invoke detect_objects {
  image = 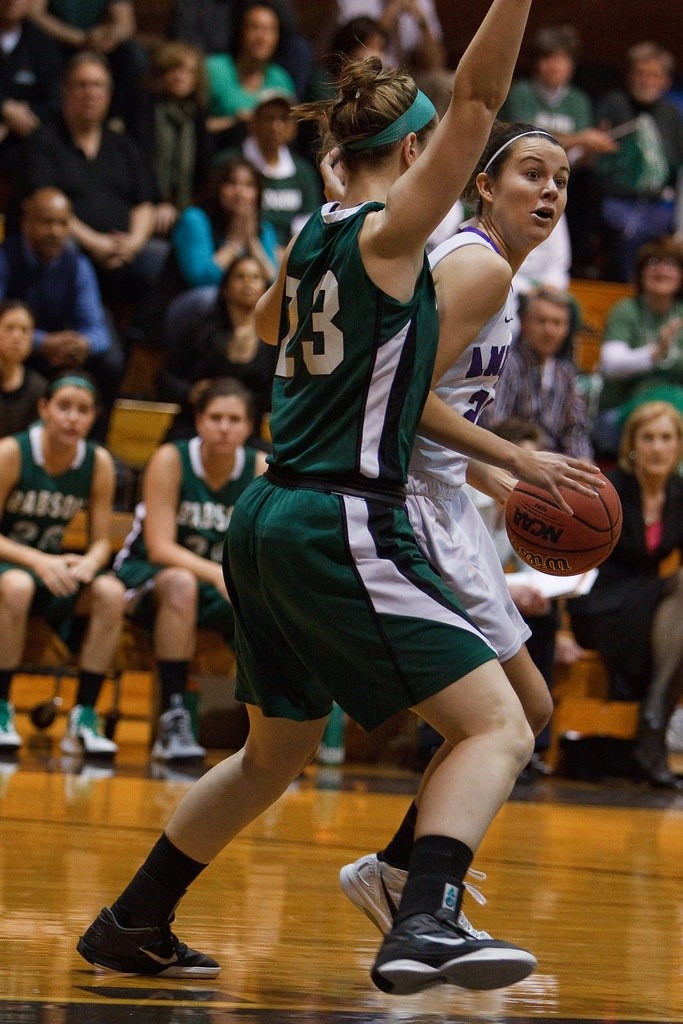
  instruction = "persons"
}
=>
[1,1,682,776]
[339,122,604,946]
[76,1,538,996]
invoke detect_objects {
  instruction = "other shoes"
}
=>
[628,709,676,789]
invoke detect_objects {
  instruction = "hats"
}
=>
[255,88,298,114]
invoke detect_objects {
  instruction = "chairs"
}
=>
[0,399,235,679]
[540,647,640,774]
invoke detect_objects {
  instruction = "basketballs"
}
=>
[505,464,622,578]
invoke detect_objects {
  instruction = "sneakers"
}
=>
[370,909,537,995]
[60,704,118,757]
[77,907,222,979]
[339,854,495,941]
[150,708,208,764]
[0,702,23,754]
